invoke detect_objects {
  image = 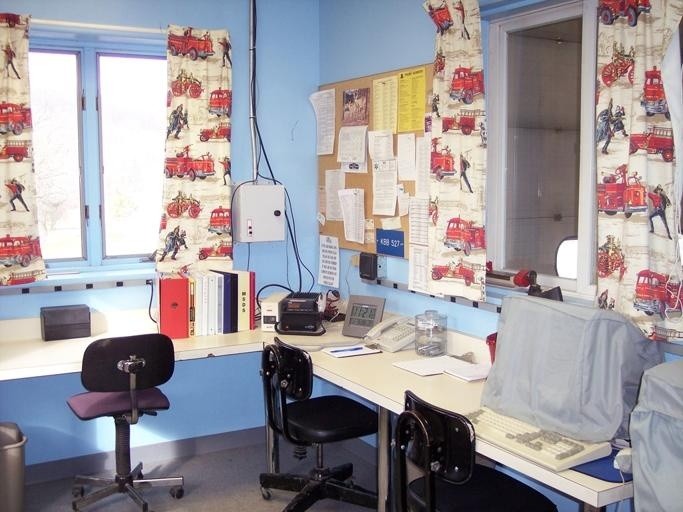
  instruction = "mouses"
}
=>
[614,447,633,472]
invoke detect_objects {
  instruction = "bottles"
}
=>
[415,309,447,356]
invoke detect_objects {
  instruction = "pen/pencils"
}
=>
[331,347,363,353]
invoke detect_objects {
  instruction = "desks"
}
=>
[0,293,632,512]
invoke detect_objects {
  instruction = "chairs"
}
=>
[67,332,186,511]
[254,329,377,512]
[391,384,561,512]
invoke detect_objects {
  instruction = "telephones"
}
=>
[364,316,416,353]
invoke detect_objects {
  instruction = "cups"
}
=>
[487,332,497,363]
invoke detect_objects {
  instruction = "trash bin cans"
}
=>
[0,422,27,512]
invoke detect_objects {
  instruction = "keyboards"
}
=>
[462,406,612,472]
